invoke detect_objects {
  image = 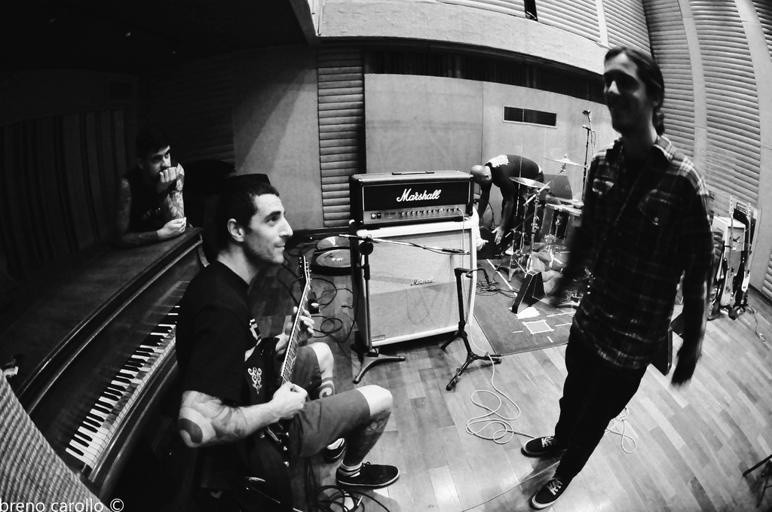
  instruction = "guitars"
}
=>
[239,254,311,469]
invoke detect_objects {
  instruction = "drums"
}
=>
[540,201,581,241]
[544,197,582,209]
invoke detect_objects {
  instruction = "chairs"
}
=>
[158,379,364,512]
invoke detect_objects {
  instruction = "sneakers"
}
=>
[335,462,399,488]
[323,439,345,463]
[531,478,569,509]
[523,435,567,456]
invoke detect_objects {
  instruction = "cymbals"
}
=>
[510,177,550,191]
[553,157,583,168]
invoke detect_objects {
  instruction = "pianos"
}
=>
[0,225,209,512]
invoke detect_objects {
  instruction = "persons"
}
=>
[470,153,544,245]
[111,130,191,248]
[175,175,400,494]
[519,46,713,509]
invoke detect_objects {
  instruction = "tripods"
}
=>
[441,268,501,390]
[494,164,566,282]
[339,234,470,383]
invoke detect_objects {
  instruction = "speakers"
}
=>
[354,207,480,349]
[525,175,572,242]
[350,170,474,230]
